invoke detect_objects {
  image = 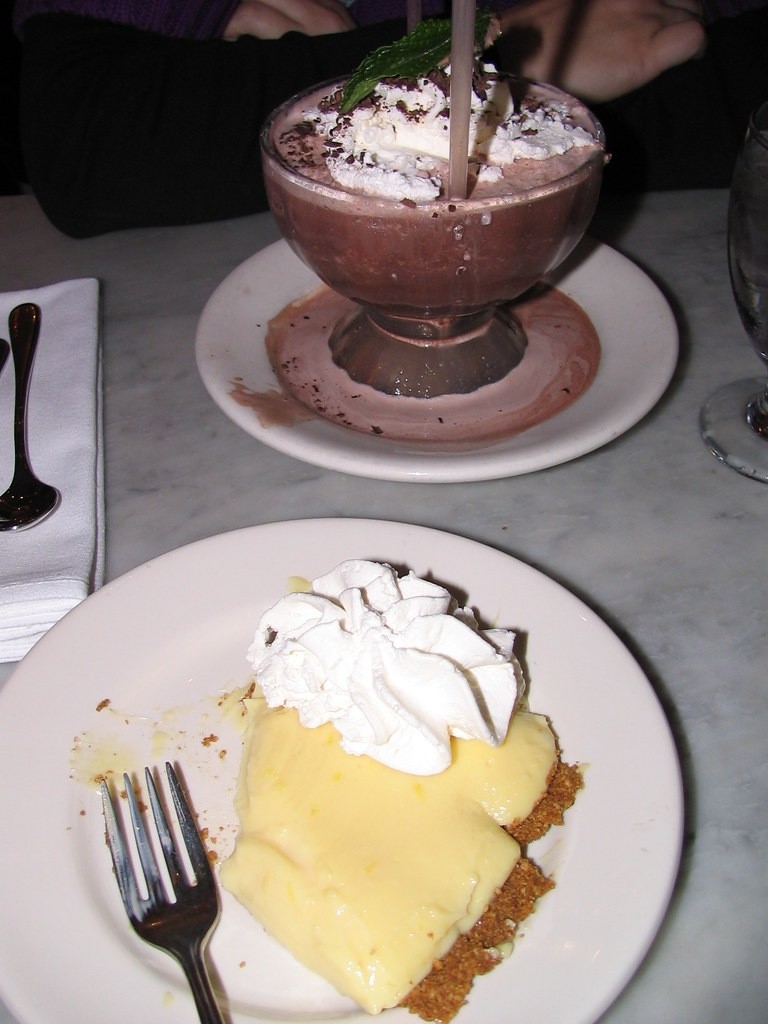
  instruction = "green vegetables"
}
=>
[336,7,500,114]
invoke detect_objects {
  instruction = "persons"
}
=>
[10,0,768,239]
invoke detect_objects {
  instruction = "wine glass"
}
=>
[698,95,768,483]
[258,70,609,391]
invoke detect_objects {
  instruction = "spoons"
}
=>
[0,303,60,532]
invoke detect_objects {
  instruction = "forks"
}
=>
[102,761,222,1024]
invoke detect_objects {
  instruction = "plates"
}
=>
[0,519,684,1024]
[195,237,680,471]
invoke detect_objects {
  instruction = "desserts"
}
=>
[258,54,608,313]
[217,562,586,1024]
